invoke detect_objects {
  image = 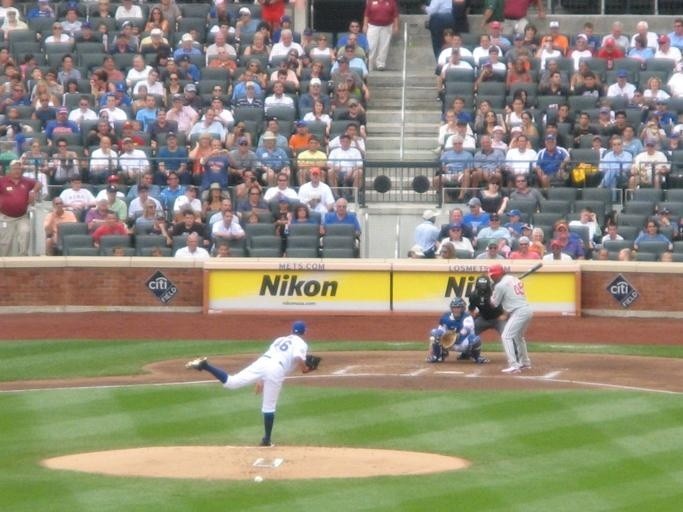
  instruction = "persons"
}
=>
[424,296,489,363]
[185,321,322,447]
[488,263,534,373]
[456,275,519,363]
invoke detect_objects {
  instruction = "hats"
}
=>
[578,34,681,215]
[474,275,490,286]
[290,320,307,336]
[6,5,365,206]
[422,197,568,252]
[486,264,505,277]
[452,21,560,139]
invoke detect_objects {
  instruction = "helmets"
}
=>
[447,297,467,308]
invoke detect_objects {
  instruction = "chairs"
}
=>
[436,31,683,260]
[0,0,367,259]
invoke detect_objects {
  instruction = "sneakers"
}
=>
[183,355,209,372]
[519,361,531,369]
[498,365,522,374]
[442,352,449,358]
[473,356,490,363]
[262,437,272,446]
[454,353,469,360]
[424,355,443,363]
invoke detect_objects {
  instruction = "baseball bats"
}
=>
[517,263,542,279]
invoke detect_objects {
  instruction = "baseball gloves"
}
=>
[441,330,456,349]
[306,354,321,369]
